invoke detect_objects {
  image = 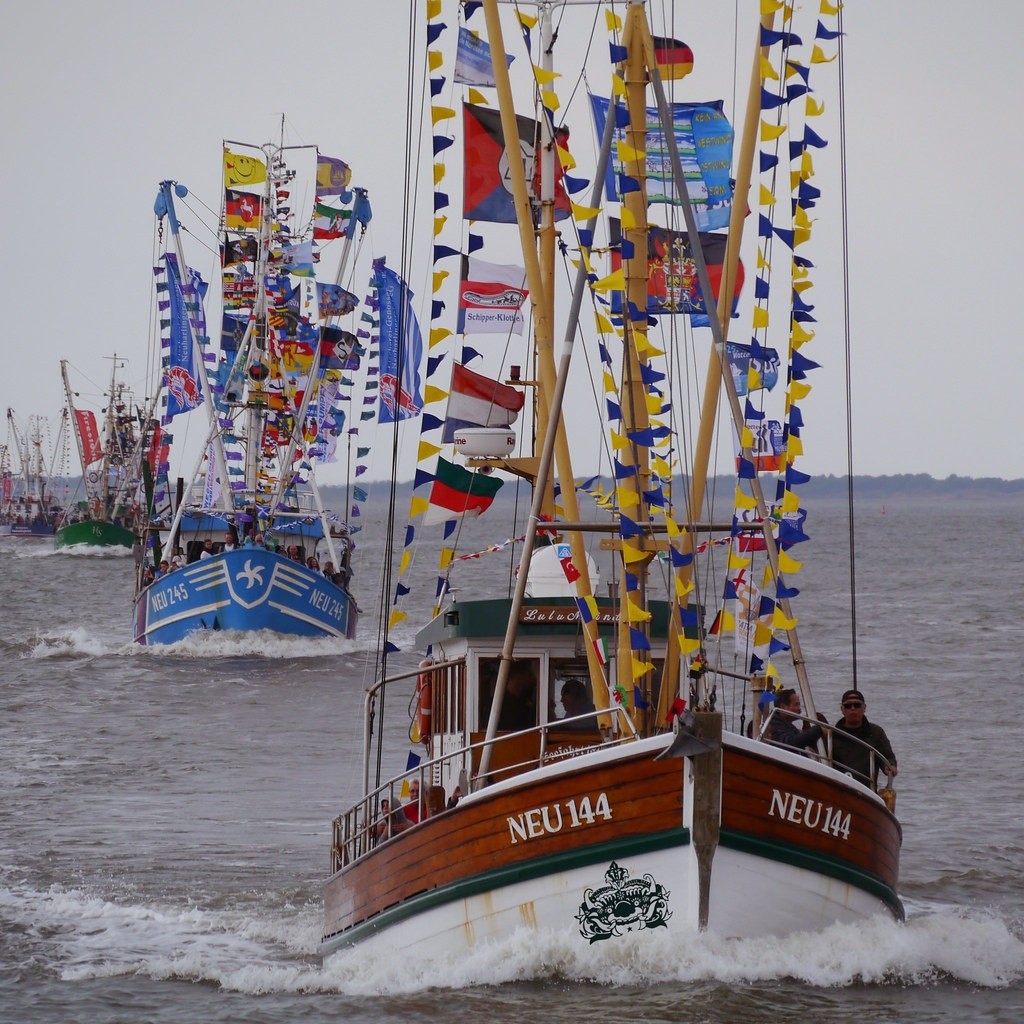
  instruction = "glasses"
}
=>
[844,703,861,709]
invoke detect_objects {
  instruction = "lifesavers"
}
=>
[415,661,434,744]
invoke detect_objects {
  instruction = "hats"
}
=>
[172,556,181,563]
[339,567,345,572]
[841,690,864,703]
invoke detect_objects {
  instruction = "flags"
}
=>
[143,144,388,577]
[453,27,516,88]
[732,569,752,591]
[164,251,208,417]
[459,251,529,334]
[560,556,582,582]
[74,403,172,526]
[630,625,650,650]
[420,457,505,527]
[590,91,734,237]
[608,214,747,316]
[593,635,610,665]
[4,479,12,504]
[715,339,786,660]
[375,262,421,423]
[578,595,600,622]
[461,100,575,222]
[665,697,687,724]
[442,362,527,446]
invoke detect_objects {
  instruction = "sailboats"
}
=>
[0,350,163,567]
[317,0,907,1009]
[131,110,423,664]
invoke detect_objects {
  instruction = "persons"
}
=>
[140,530,345,586]
[825,689,897,793]
[555,679,599,731]
[747,688,828,758]
[16,513,31,525]
[69,514,79,524]
[370,777,462,849]
